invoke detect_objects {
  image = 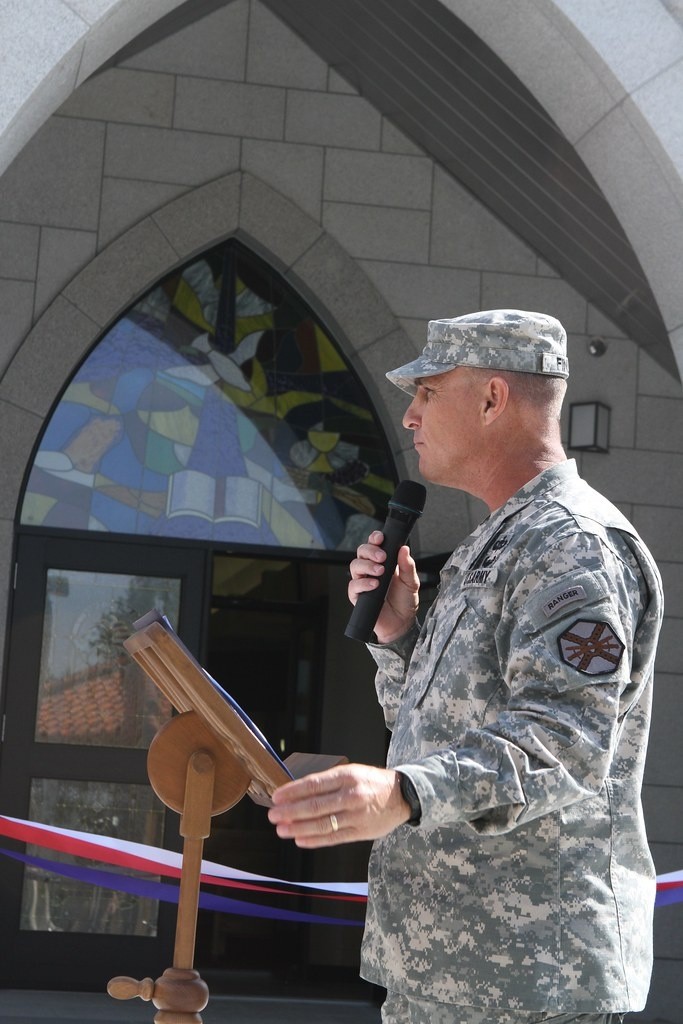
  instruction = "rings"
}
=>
[329,813,340,831]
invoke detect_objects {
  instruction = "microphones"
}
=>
[344,479,426,643]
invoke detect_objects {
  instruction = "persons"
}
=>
[270,308,664,1024]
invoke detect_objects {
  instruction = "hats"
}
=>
[384,309,570,398]
